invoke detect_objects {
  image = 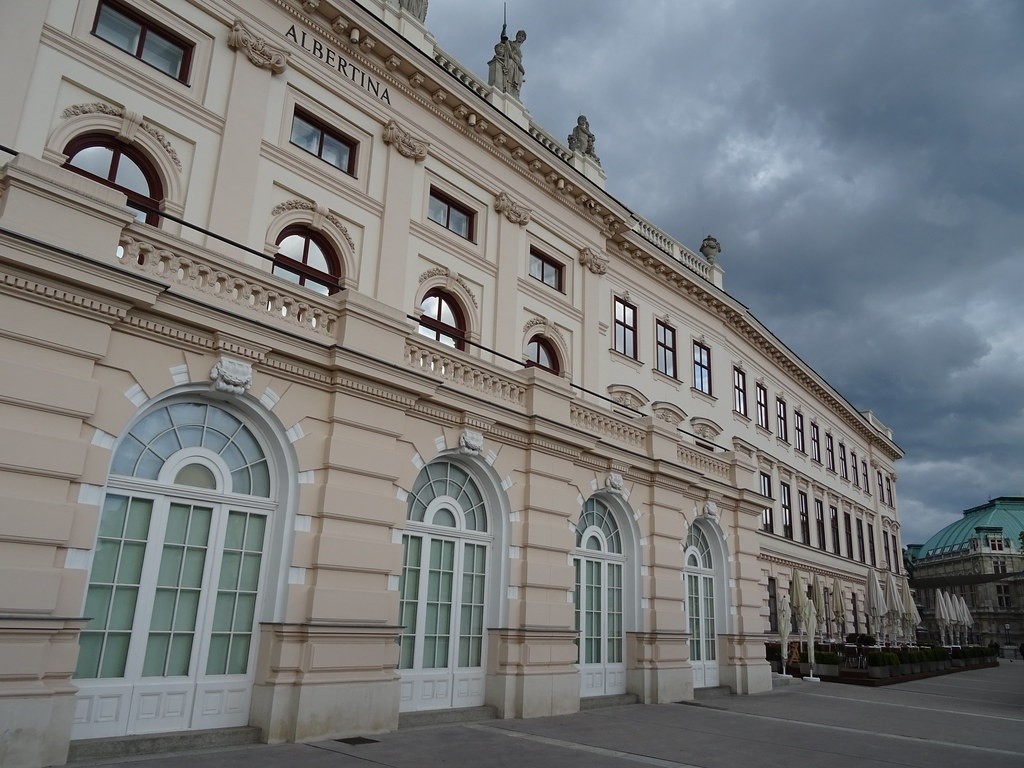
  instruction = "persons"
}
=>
[988,640,1000,657]
[1019,643,1024,660]
[573,115,595,156]
[499,23,526,94]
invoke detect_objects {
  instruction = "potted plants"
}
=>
[797,651,839,676]
[867,645,998,678]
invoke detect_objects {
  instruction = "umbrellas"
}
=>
[778,568,975,684]
